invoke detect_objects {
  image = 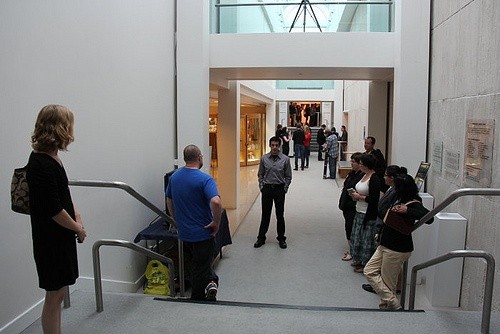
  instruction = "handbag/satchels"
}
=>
[382,200,422,236]
[10,164,30,214]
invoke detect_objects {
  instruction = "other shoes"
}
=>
[341,251,352,260]
[293,169,298,170]
[351,261,363,272]
[326,177,335,179]
[305,166,308,168]
[318,159,324,161]
[362,284,375,292]
[301,169,304,170]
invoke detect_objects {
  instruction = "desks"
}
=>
[139,207,232,259]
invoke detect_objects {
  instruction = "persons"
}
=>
[276,124,282,138]
[303,125,311,168]
[293,123,305,170]
[361,165,407,294]
[362,136,387,173]
[339,152,364,261]
[254,136,292,248]
[165,144,221,301]
[349,154,381,273]
[322,129,338,179]
[280,127,291,156]
[317,125,326,161]
[26,104,87,334]
[338,126,347,161]
[363,174,435,308]
[289,102,320,127]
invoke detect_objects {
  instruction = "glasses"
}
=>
[384,174,388,176]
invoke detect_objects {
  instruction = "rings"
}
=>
[77,224,83,233]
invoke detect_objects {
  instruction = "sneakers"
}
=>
[205,281,218,301]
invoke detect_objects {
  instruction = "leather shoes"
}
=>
[254,236,266,248]
[279,241,287,248]
